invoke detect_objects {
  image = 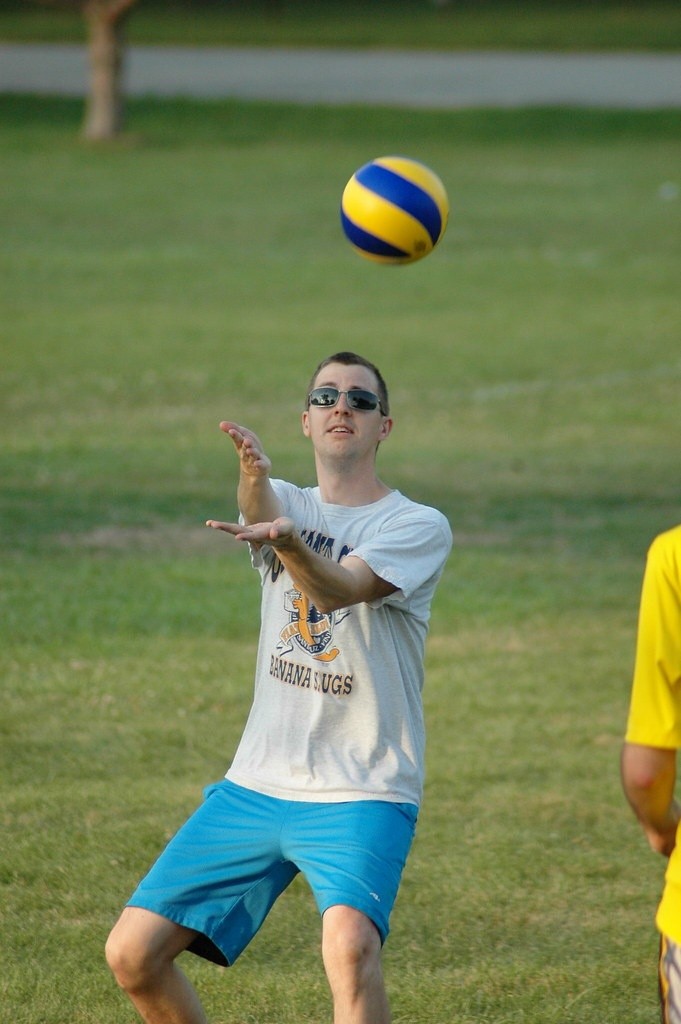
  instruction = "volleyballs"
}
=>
[341,155,451,266]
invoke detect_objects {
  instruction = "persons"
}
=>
[105,352,453,1024]
[620,522,681,1024]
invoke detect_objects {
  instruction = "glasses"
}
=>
[307,386,387,416]
